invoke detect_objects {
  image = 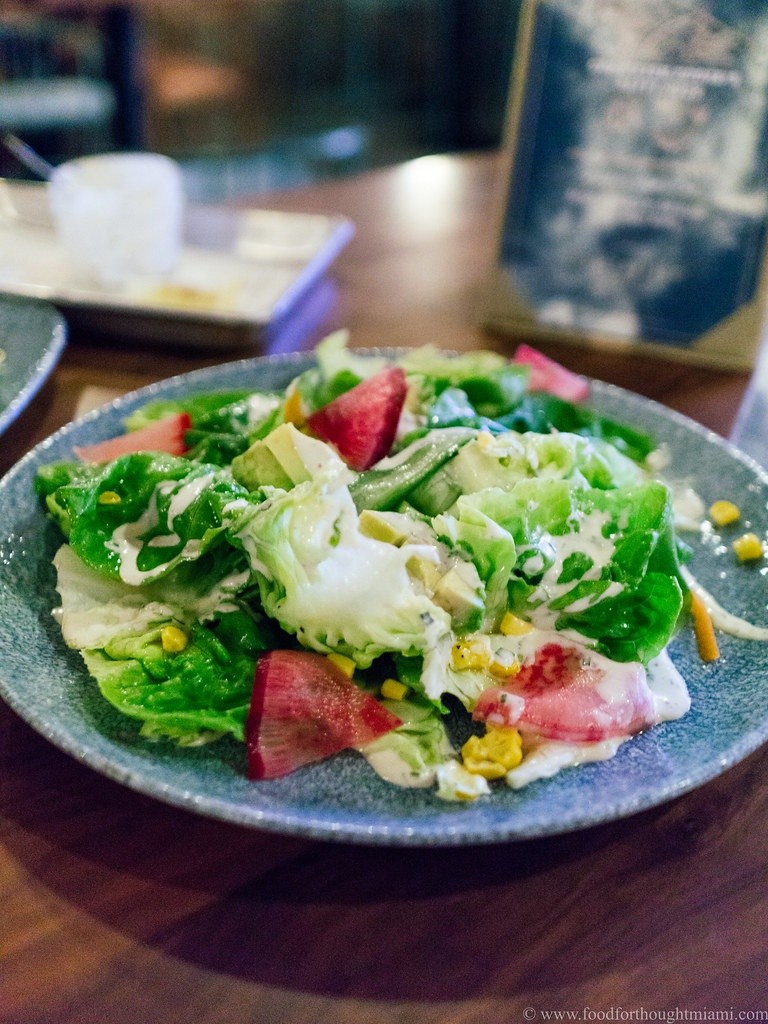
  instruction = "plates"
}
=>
[0,177,357,347]
[0,347,768,847]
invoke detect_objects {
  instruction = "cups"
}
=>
[49,152,184,284]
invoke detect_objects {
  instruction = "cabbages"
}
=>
[39,334,686,743]
